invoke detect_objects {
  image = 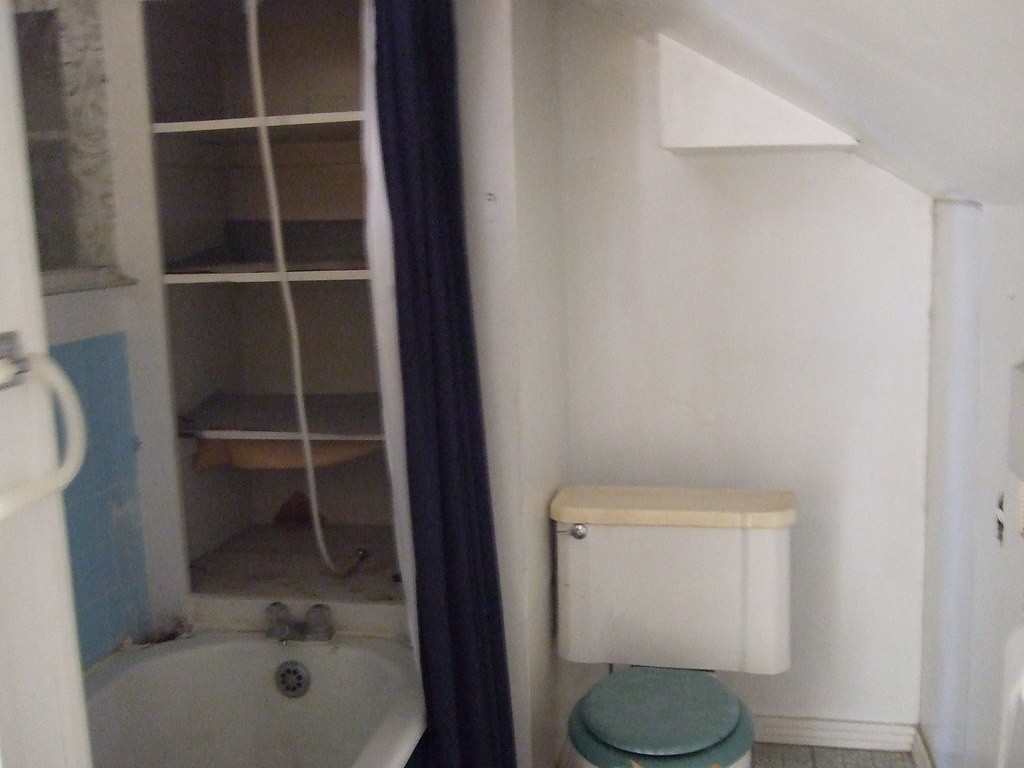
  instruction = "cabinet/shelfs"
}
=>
[100,0,411,638]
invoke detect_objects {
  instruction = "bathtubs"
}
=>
[82,629,426,767]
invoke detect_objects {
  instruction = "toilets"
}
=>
[548,483,804,768]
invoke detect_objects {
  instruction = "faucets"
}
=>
[263,601,336,645]
